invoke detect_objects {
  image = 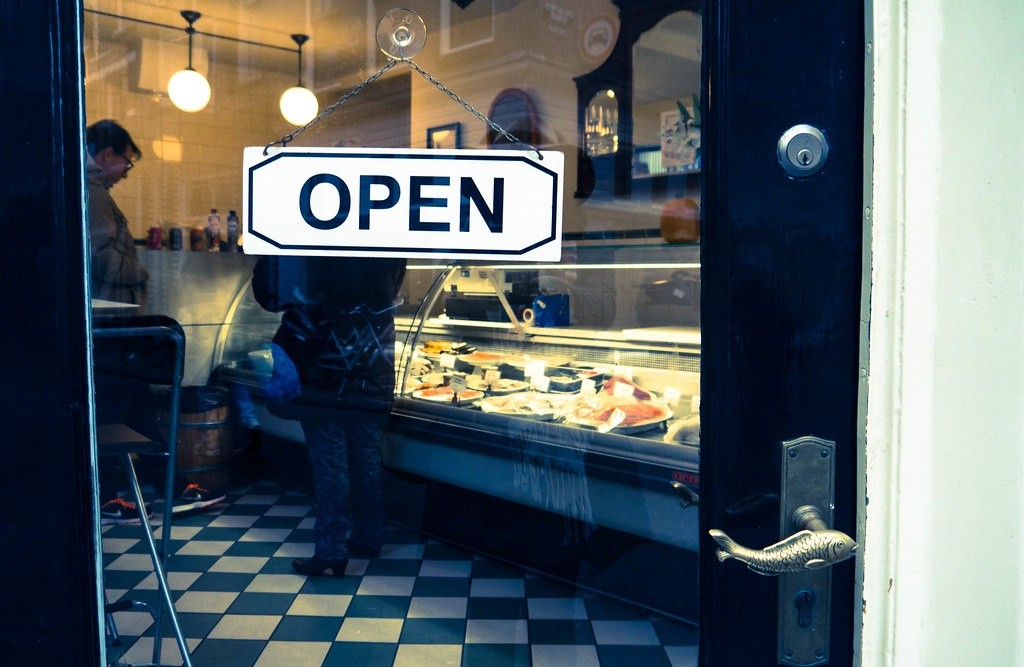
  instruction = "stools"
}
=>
[150,385,232,476]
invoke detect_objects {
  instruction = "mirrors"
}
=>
[572,0,703,201]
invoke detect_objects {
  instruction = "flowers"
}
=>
[658,94,701,156]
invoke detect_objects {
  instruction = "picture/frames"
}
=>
[426,122,462,149]
[486,87,539,149]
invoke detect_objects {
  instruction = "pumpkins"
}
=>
[660,191,700,244]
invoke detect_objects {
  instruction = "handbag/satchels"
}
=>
[272,310,328,380]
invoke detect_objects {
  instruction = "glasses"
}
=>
[121,154,134,172]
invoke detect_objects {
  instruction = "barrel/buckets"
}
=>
[158,386,232,497]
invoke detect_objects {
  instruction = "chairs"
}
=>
[92,314,193,667]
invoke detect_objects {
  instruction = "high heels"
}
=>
[292,555,349,578]
[344,541,356,556]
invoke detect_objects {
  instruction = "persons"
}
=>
[251,138,407,576]
[87,118,226,524]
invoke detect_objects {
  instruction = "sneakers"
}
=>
[172,483,226,514]
[100,497,153,525]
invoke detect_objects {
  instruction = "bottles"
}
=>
[207,209,220,252]
[226,209,238,253]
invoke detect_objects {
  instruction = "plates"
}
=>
[395,357,674,435]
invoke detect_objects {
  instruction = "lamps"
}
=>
[278,33,319,127]
[167,10,211,113]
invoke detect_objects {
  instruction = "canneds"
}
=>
[148,227,162,249]
[168,227,182,249]
[189,229,204,250]
